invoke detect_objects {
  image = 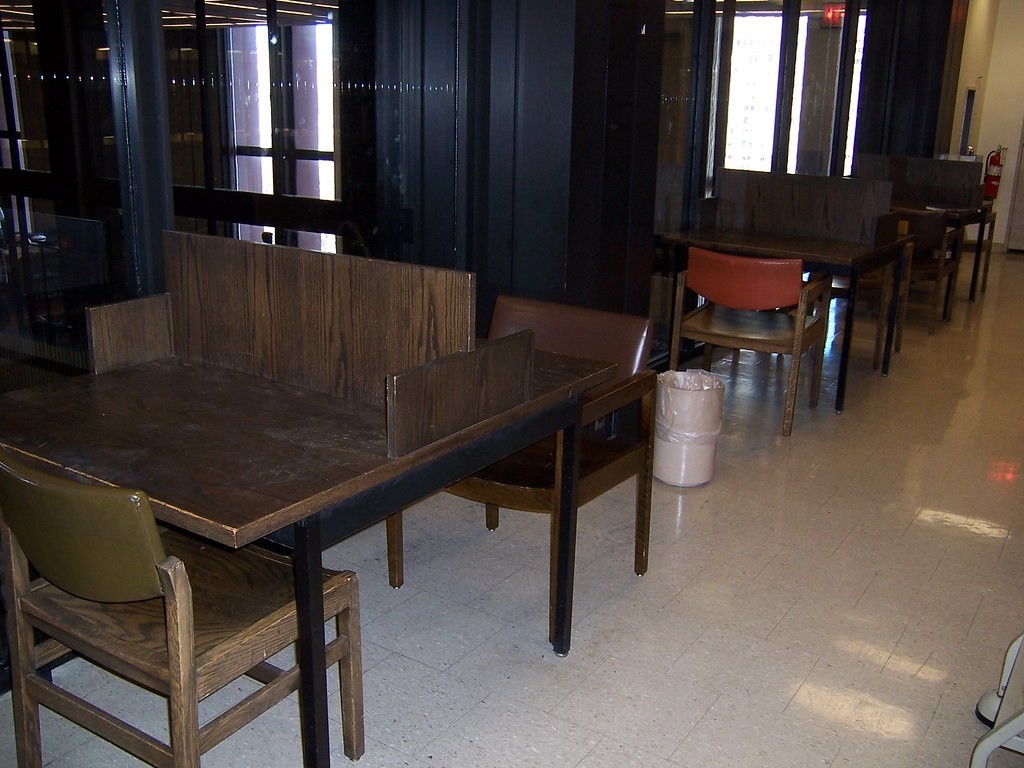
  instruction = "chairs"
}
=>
[668,246,831,436]
[807,220,915,371]
[386,295,658,657]
[0,448,364,768]
[867,208,958,336]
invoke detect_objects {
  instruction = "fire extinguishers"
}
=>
[984,144,1008,199]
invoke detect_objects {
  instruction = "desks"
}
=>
[663,168,916,415]
[848,151,988,323]
[0,228,619,768]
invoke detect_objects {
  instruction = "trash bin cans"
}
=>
[653,369,725,489]
